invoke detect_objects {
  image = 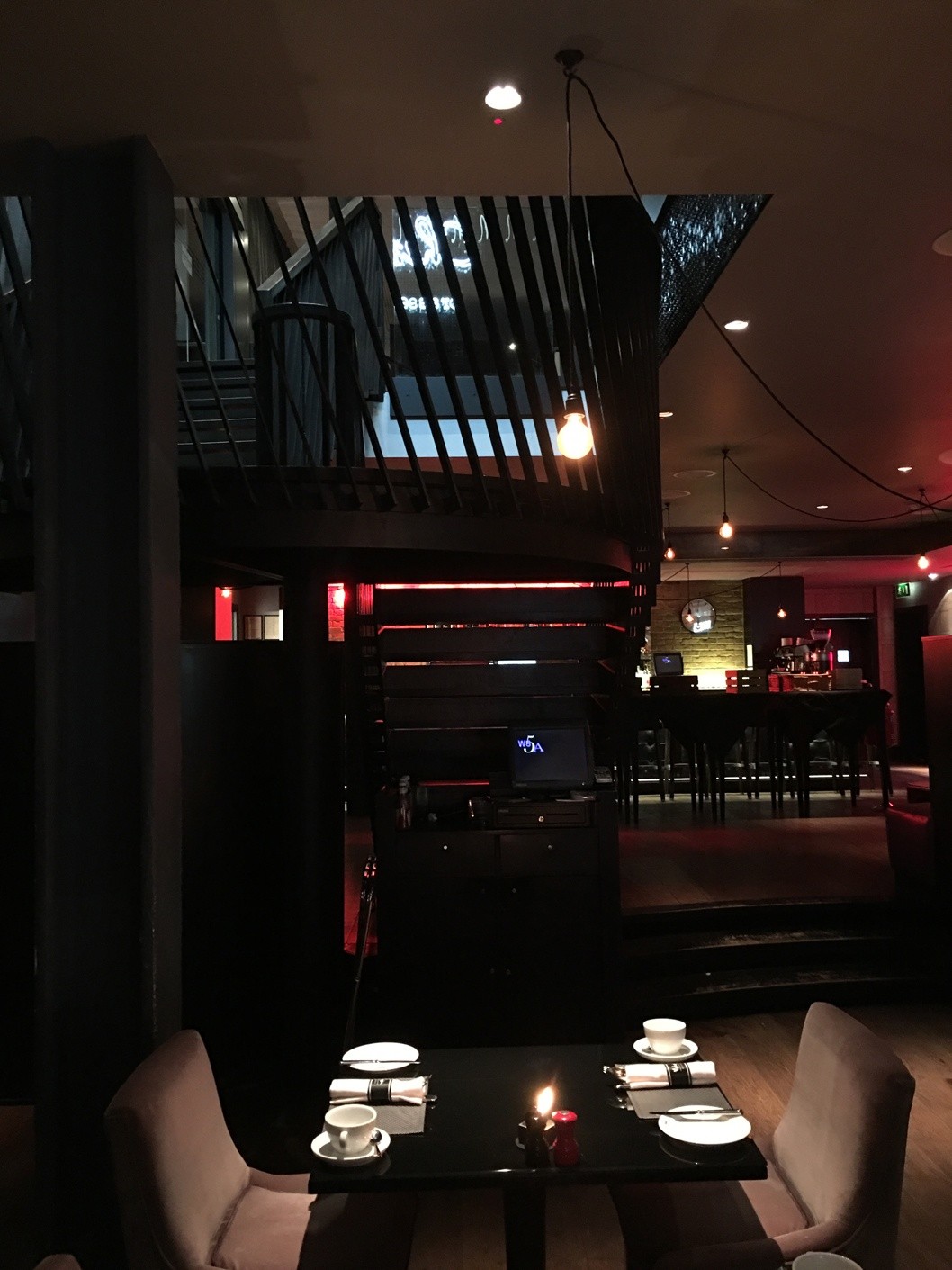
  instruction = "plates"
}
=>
[342,1042,420,1074]
[659,1133,752,1166]
[633,1037,698,1063]
[311,1127,391,1167]
[658,1105,752,1145]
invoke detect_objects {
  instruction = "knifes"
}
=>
[649,1109,744,1115]
[340,1060,421,1065]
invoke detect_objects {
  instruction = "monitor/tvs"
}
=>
[653,652,683,676]
[508,726,595,788]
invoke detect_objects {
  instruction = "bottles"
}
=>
[523,1111,579,1166]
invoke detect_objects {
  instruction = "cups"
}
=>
[324,1104,377,1154]
[643,1018,686,1055]
[792,1251,863,1270]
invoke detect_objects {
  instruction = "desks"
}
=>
[593,689,893,813]
[307,980,765,1270]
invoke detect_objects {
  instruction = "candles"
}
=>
[517,1085,555,1147]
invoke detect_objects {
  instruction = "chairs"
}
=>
[608,1001,915,1270]
[104,1029,317,1270]
[597,677,860,826]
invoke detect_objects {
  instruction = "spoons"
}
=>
[605,1095,627,1109]
[607,1068,626,1077]
[371,1130,383,1158]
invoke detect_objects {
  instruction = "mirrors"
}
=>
[681,597,716,633]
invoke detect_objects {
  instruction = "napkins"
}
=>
[329,1076,429,1104]
[602,1061,717,1090]
